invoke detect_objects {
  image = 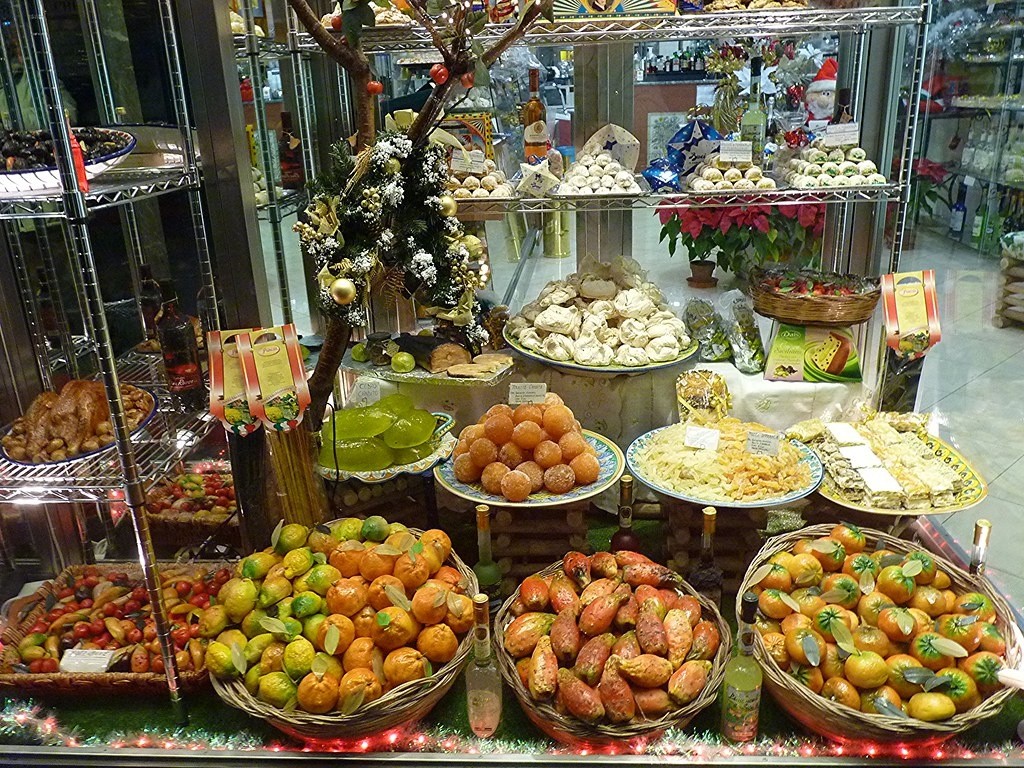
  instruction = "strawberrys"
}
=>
[759,277,854,296]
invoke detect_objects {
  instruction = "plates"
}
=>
[433,431,625,508]
[502,324,703,379]
[132,347,204,358]
[0,387,159,466]
[313,413,458,483]
[625,426,824,507]
[0,127,137,192]
[816,433,989,515]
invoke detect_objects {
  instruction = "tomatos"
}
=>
[25,568,230,674]
[150,474,235,512]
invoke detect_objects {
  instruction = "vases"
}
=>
[728,241,810,280]
[886,223,917,250]
[686,259,718,288]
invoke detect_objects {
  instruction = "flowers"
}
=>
[653,193,829,275]
[889,155,952,225]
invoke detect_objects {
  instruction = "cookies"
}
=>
[790,416,965,510]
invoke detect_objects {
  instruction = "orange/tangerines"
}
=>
[753,525,1007,720]
[298,522,475,720]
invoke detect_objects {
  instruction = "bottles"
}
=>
[472,505,502,629]
[947,119,1024,254]
[689,507,722,613]
[720,592,762,744]
[156,278,206,408]
[763,97,776,170]
[968,519,992,576]
[278,110,305,191]
[466,593,502,738]
[36,270,70,346]
[523,69,548,164]
[632,44,704,82]
[740,56,766,176]
[138,264,164,341]
[610,475,640,556]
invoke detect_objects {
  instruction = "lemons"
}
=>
[200,515,414,709]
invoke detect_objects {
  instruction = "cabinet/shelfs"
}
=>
[283,0,933,432]
[0,0,226,728]
[912,0,1024,259]
